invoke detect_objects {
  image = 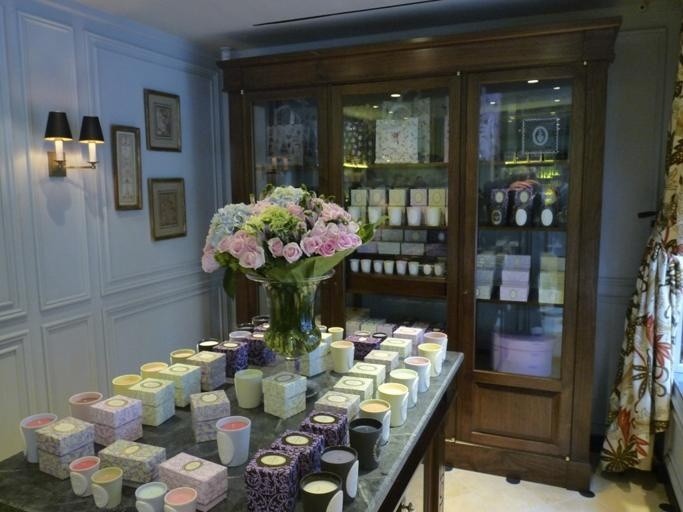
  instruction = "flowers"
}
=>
[201,181,365,349]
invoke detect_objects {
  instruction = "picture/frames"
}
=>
[148,178,188,240]
[143,87,183,152]
[110,124,143,212]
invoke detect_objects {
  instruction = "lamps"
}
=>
[41,111,104,179]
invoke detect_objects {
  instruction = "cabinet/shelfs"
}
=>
[216,16,629,500]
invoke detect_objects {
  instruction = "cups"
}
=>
[348,258,446,279]
[229,310,270,342]
[344,204,450,228]
[19,333,218,470]
[299,329,448,512]
[232,367,264,411]
[314,323,347,338]
[329,338,355,372]
[61,456,199,512]
[213,416,250,469]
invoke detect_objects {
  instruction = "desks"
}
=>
[0,330,463,512]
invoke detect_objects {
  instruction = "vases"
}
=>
[241,270,340,394]
